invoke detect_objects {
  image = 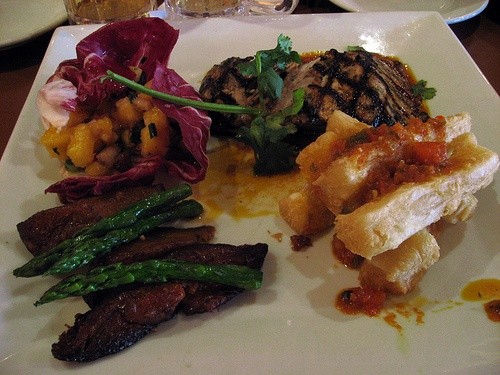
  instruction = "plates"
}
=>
[1,10,500,375]
[1,0,79,47]
[331,0,490,25]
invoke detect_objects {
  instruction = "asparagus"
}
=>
[13,188,264,308]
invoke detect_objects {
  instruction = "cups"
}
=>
[61,0,157,27]
[243,0,301,17]
[165,0,248,21]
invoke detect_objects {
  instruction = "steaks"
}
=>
[17,178,269,364]
